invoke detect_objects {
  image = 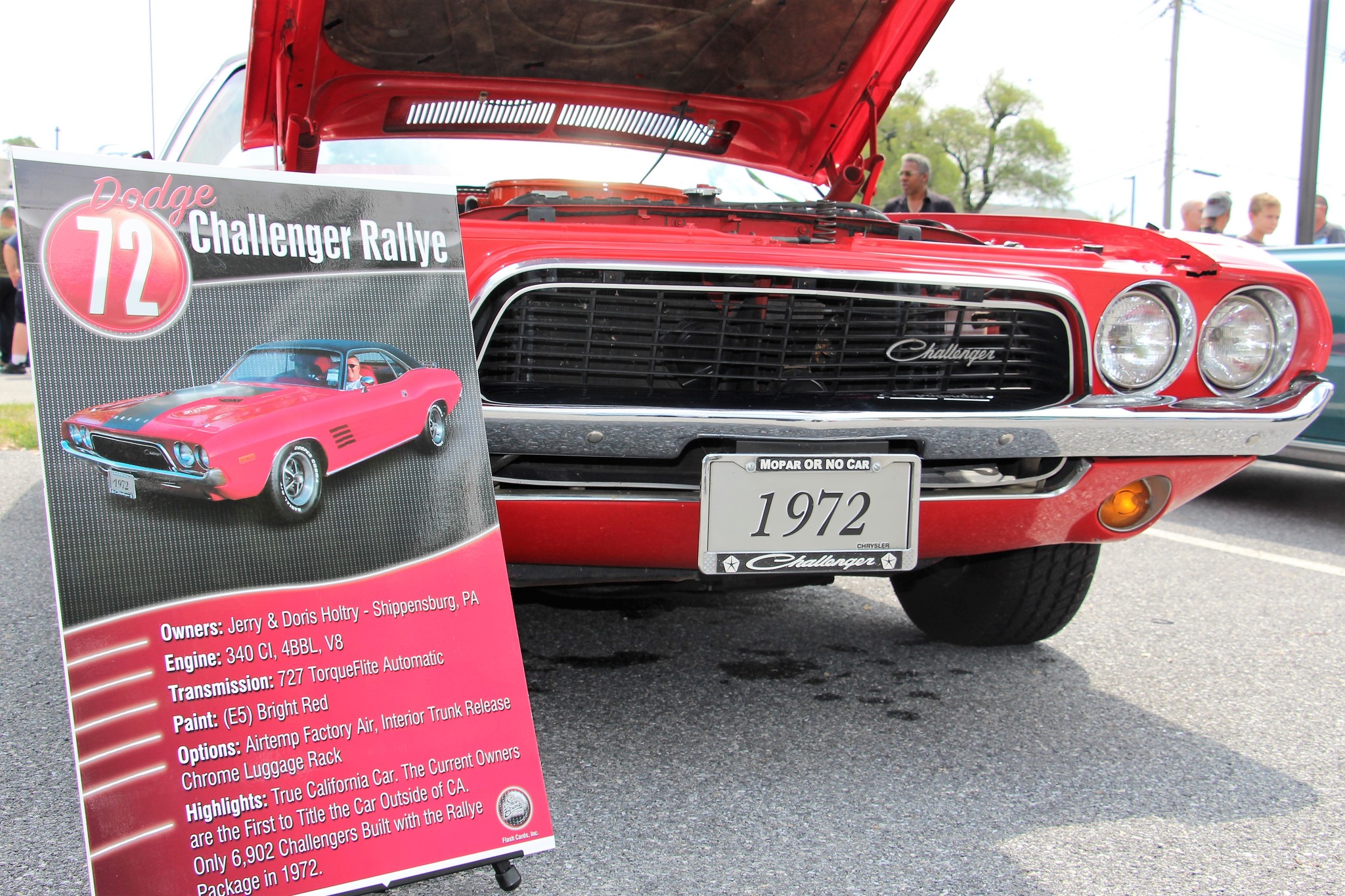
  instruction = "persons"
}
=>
[0,206,30,374]
[883,153,957,213]
[1180,191,1281,244]
[1312,195,1345,244]
[345,355,365,391]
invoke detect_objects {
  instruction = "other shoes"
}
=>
[0,364,26,374]
[20,362,30,367]
[0,356,12,366]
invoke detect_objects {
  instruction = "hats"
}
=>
[1202,192,1232,218]
[289,354,317,361]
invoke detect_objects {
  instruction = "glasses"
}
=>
[347,364,358,368]
[897,170,919,176]
[296,363,310,370]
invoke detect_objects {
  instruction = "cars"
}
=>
[235,0,1337,643]
[59,335,463,529]
[1248,240,1345,476]
